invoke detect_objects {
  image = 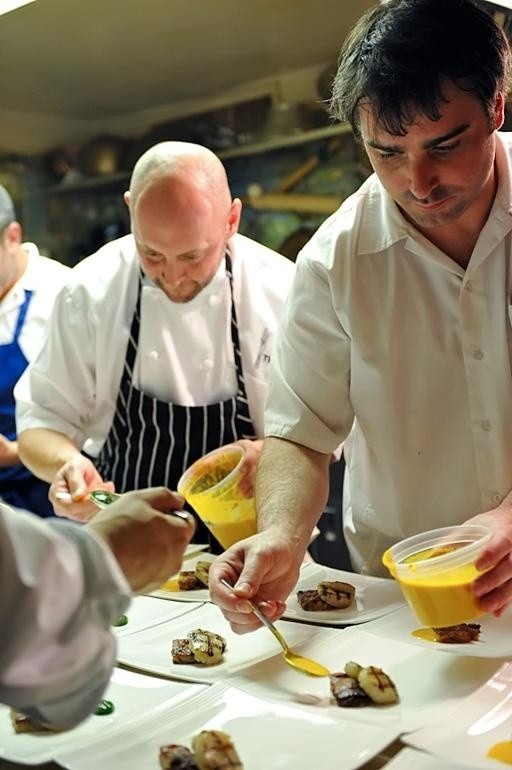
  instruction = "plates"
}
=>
[104,594,203,644]
[399,659,512,770]
[7,664,209,766]
[366,590,511,663]
[136,552,224,607]
[275,565,404,625]
[104,601,330,690]
[48,678,404,768]
[231,624,511,733]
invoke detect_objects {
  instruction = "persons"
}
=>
[206,0,510,636]
[0,186,73,522]
[12,139,343,553]
[0,485,196,733]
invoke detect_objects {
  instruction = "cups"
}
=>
[177,445,258,551]
[383,525,497,629]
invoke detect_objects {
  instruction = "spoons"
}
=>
[89,489,190,523]
[216,575,336,681]
